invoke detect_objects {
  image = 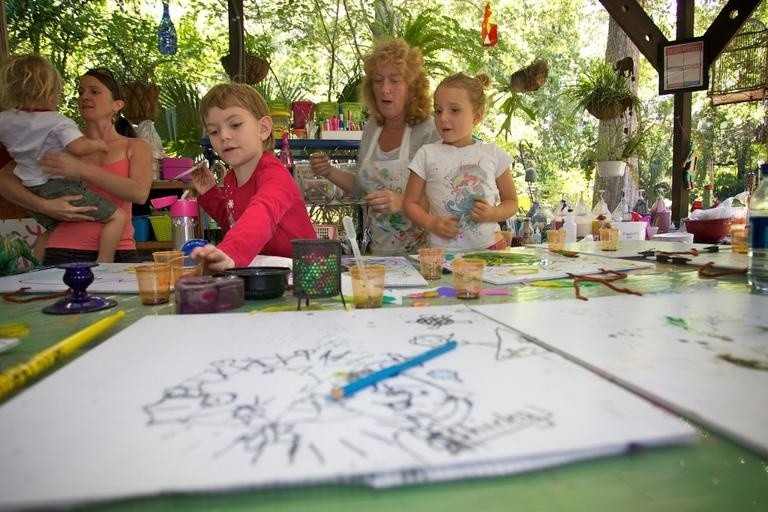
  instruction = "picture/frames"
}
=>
[657,36,709,95]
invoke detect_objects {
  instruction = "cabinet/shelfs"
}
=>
[128,179,202,263]
[201,138,363,243]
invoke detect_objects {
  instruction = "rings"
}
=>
[381,204,387,210]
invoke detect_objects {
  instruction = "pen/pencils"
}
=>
[332,341,457,401]
[174,160,205,180]
[319,105,365,131]
[0,310,126,399]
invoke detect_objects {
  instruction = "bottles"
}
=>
[171,190,200,250]
[563,209,576,247]
[748,164,768,294]
[519,217,542,245]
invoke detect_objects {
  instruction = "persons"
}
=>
[189,81,328,273]
[0,67,155,270]
[0,52,129,266]
[401,71,520,250]
[307,36,441,254]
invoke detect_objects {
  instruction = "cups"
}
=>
[600,228,620,251]
[452,259,484,299]
[495,232,513,252]
[347,266,385,308]
[418,248,445,279]
[134,250,206,305]
[547,230,567,251]
[731,224,748,254]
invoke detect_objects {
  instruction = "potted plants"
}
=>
[106,33,180,126]
[551,52,651,121]
[579,121,661,187]
[219,30,277,86]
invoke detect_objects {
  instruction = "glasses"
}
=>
[96,67,114,79]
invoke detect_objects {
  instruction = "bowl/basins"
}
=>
[683,218,732,244]
[222,267,292,300]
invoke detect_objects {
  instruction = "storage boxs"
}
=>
[132,215,153,242]
[610,221,647,240]
[147,215,174,242]
